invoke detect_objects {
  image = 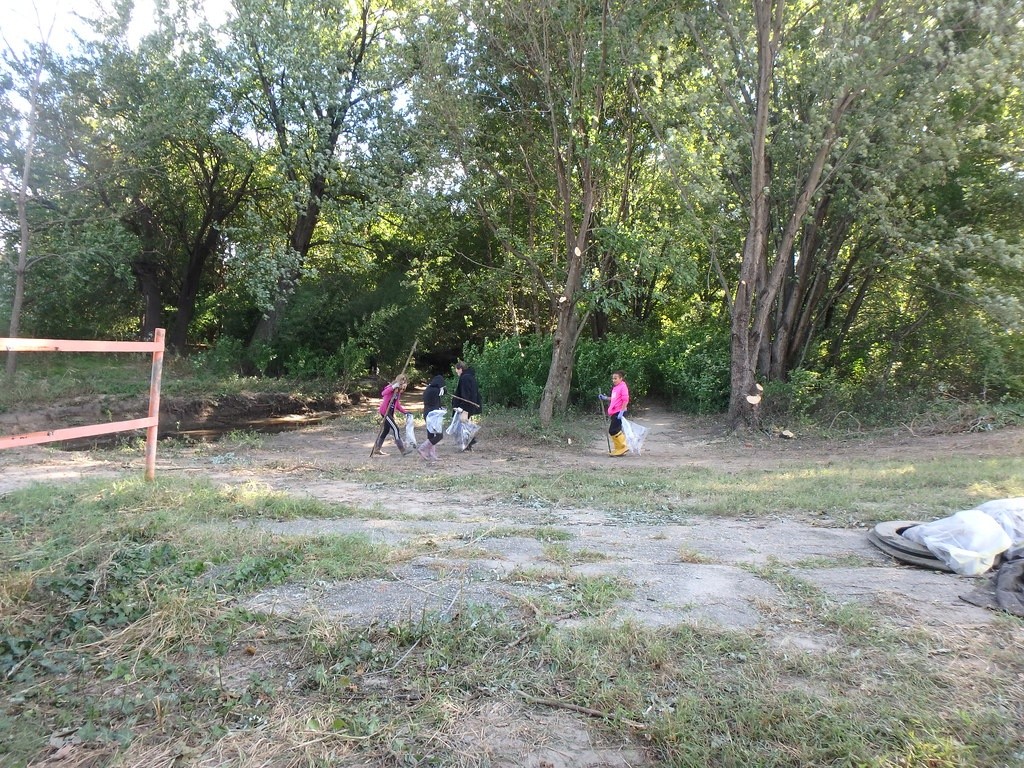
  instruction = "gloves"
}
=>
[598,393,608,401]
[617,410,624,419]
[454,407,463,413]
[438,387,444,396]
[392,383,400,390]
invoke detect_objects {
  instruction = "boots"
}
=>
[395,439,413,456]
[374,438,387,454]
[607,430,629,457]
[417,439,433,461]
[428,445,444,461]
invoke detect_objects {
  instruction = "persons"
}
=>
[417,375,448,461]
[373,375,413,458]
[599,370,631,457]
[452,361,482,452]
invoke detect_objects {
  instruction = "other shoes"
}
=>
[463,437,476,453]
